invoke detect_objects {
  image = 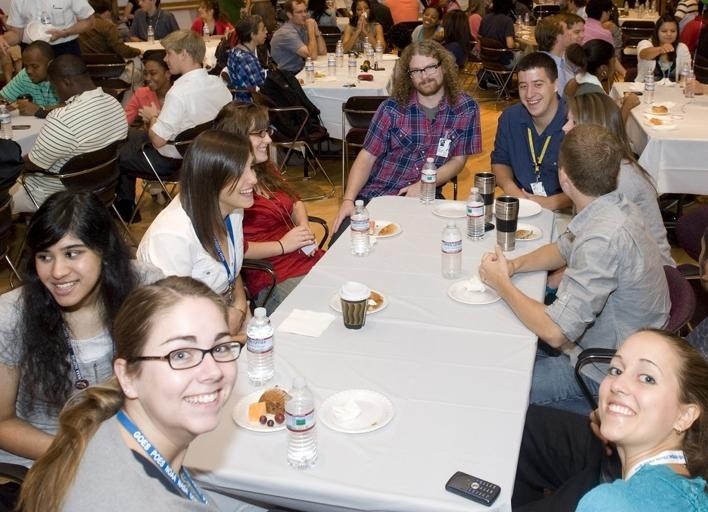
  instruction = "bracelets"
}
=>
[230,304,248,319]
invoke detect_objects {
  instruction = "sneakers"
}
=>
[470,49,478,57]
[476,52,481,59]
[277,152,297,166]
[476,71,488,90]
[487,76,509,88]
[312,140,340,153]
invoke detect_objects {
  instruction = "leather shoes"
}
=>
[109,198,141,224]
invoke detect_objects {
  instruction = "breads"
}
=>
[651,118,663,124]
[378,224,398,234]
[260,388,291,415]
[368,292,382,309]
[652,105,667,112]
[515,230,533,238]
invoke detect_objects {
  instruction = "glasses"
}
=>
[408,60,443,79]
[247,126,274,138]
[126,338,245,370]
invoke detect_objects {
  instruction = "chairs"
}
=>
[123,118,217,241]
[573,264,697,412]
[341,96,392,195]
[387,21,422,47]
[241,212,329,317]
[79,53,136,99]
[436,174,458,199]
[689,5,707,80]
[619,19,656,67]
[21,139,138,248]
[473,47,521,100]
[227,83,334,189]
[320,25,343,52]
[0,188,24,282]
[534,5,560,19]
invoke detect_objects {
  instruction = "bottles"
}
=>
[516,13,541,31]
[41,10,53,38]
[0,105,13,139]
[304,39,382,85]
[614,0,657,18]
[203,22,210,43]
[16,94,33,114]
[246,308,317,471]
[420,157,486,277]
[644,62,696,104]
[350,199,369,256]
[147,24,155,45]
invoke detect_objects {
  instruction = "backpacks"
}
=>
[259,68,324,139]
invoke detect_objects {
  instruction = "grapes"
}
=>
[267,421,274,425]
[274,414,284,422]
[260,417,269,425]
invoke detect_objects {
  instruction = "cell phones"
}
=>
[623,92,643,96]
[445,470,501,507]
[11,125,31,130]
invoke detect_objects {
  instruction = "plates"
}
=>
[641,107,679,131]
[329,288,386,315]
[366,218,401,238]
[516,31,531,42]
[233,388,393,432]
[432,196,543,305]
[383,54,400,61]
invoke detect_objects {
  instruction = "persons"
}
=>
[558,92,678,267]
[574,327,707,511]
[0,0,386,215]
[511,317,706,512]
[326,40,484,250]
[135,128,253,346]
[0,188,166,512]
[478,123,674,416]
[489,52,574,211]
[410,0,708,139]
[212,99,325,318]
[12,274,238,512]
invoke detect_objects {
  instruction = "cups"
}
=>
[340,282,370,330]
[474,172,519,252]
